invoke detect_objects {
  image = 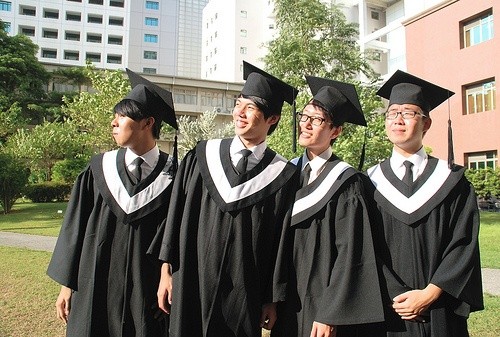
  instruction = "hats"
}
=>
[241,59,299,154]
[123,68,179,177]
[376,70,456,169]
[304,75,368,173]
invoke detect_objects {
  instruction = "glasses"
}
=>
[385,110,430,120]
[297,112,325,126]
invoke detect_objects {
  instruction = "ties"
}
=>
[300,163,311,187]
[236,149,252,174]
[401,161,414,185]
[130,157,145,181]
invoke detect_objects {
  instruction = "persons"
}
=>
[363,70,485,337]
[155,61,299,337]
[270,74,384,337]
[46,66,182,337]
